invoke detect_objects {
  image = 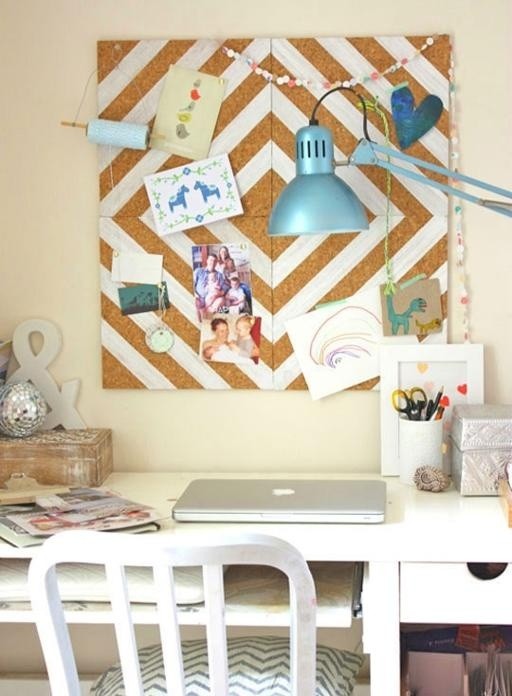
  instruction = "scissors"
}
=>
[393,388,426,421]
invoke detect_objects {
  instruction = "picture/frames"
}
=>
[379,343,485,477]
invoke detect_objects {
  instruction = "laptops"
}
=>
[171,479,387,524]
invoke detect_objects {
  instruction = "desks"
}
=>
[0,473,512,696]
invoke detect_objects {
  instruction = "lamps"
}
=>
[266,85,512,238]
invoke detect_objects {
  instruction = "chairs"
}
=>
[26,529,366,696]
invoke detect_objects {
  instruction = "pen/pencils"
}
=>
[425,386,444,421]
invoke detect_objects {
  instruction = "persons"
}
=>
[192,246,261,361]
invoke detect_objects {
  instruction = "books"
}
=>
[0,484,160,549]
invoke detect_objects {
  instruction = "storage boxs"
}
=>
[0,430,114,487]
